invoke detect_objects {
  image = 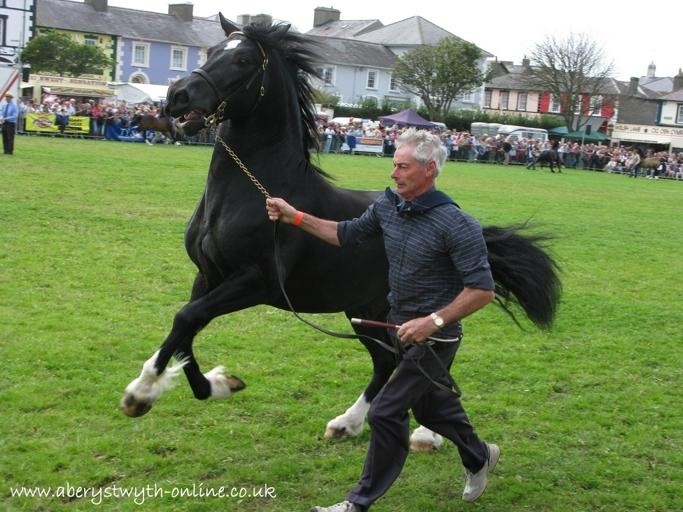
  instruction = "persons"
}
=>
[0,94,17,154]
[265,129,502,510]
[13,95,682,182]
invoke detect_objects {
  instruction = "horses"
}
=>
[129,113,180,146]
[628,144,666,179]
[119,12,568,452]
[526,139,568,173]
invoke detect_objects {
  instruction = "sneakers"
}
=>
[311,501,364,512]
[461,443,500,502]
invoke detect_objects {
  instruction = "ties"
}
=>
[3,104,8,117]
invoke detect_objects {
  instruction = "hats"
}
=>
[5,93,13,98]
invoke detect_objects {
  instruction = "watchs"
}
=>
[430,312,445,329]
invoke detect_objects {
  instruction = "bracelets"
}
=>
[291,210,304,225]
